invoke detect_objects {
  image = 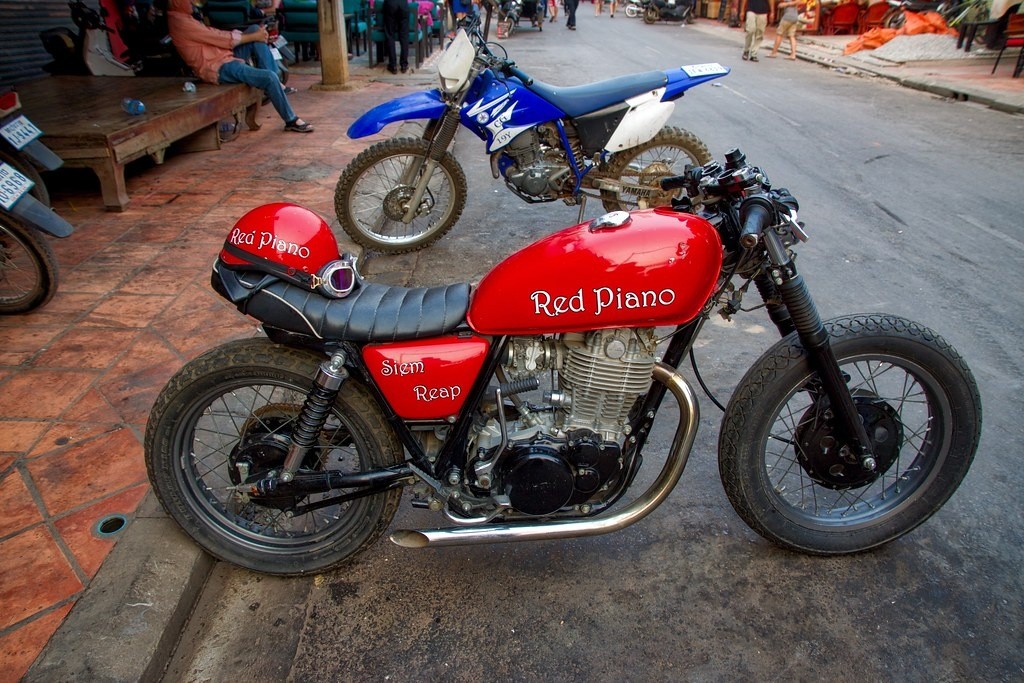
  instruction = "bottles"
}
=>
[120,97,146,115]
[218,121,239,131]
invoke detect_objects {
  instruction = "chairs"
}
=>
[857,1,890,33]
[819,1,858,36]
[958,2,1021,53]
[991,13,1024,79]
[201,0,444,69]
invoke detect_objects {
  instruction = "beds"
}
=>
[14,71,265,213]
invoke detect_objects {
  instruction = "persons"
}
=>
[564,0,579,30]
[742,0,769,62]
[202,0,264,25]
[976,0,1023,55]
[595,0,602,16]
[610,0,618,18]
[765,0,802,60]
[168,0,313,132]
[549,0,561,23]
[383,0,472,74]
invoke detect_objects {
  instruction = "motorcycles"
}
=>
[0,162,73,314]
[334,24,737,255]
[145,151,983,576]
[497,0,545,37]
[0,91,61,228]
[643,0,697,25]
[625,0,652,18]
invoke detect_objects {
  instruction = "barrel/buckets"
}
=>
[497,21,510,39]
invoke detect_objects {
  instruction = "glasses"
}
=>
[317,254,363,298]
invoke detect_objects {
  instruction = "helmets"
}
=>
[219,202,339,300]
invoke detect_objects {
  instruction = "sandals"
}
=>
[285,116,315,132]
[284,86,297,96]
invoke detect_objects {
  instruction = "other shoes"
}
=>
[765,54,776,58]
[784,56,795,60]
[751,57,759,62]
[569,25,576,30]
[742,52,748,60]
[401,67,406,72]
[387,66,397,74]
